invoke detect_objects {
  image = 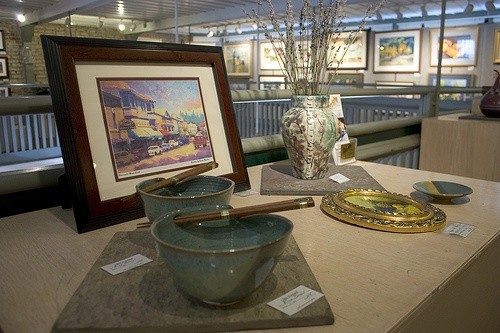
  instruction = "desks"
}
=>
[419,114,500,183]
[0,160,500,333]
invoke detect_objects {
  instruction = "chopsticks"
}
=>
[120,161,218,202]
[137,197,315,227]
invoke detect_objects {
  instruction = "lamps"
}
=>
[97,15,148,31]
[65,18,71,28]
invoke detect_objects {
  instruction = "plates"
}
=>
[412,181,474,200]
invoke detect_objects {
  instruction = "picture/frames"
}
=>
[426,72,474,115]
[228,80,250,91]
[372,29,423,74]
[325,29,369,71]
[328,73,364,89]
[429,26,480,67]
[0,57,9,79]
[258,42,287,71]
[375,80,415,117]
[0,87,11,97]
[0,30,6,52]
[222,41,254,78]
[40,35,251,234]
[258,75,287,90]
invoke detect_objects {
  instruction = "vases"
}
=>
[280,95,340,180]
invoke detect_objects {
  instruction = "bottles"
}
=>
[479,73,500,118]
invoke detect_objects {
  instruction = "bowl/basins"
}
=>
[150,204,294,305]
[136,177,235,221]
